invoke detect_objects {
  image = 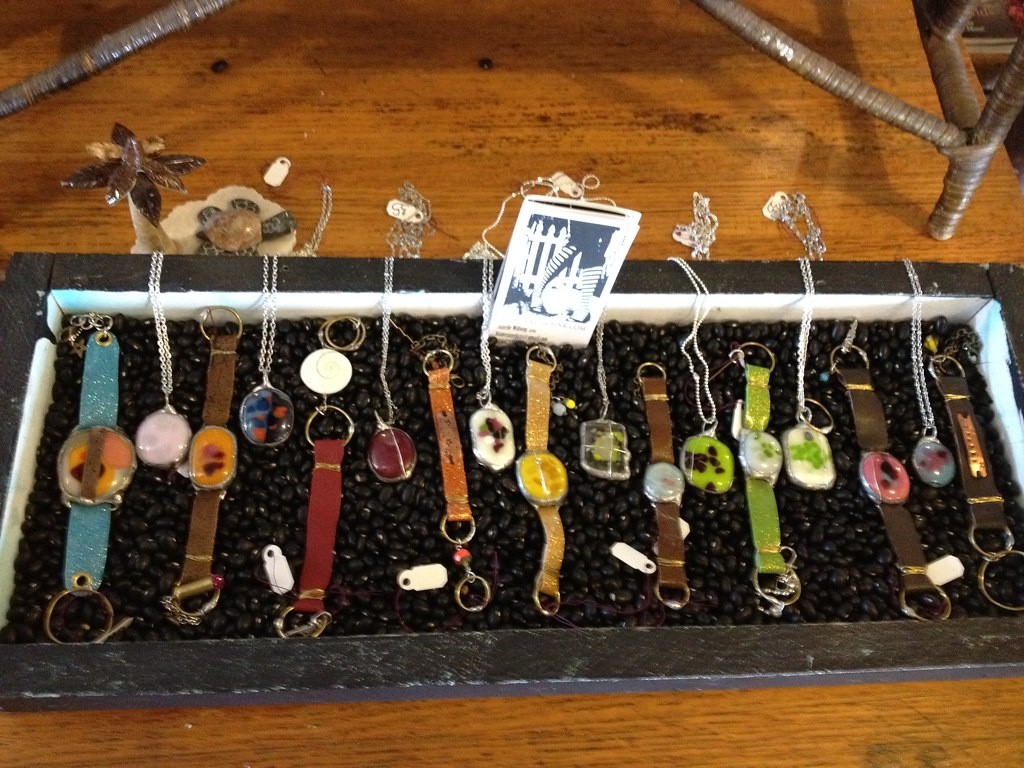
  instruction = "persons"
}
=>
[529,221,615,322]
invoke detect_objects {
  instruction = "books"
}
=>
[488,196,642,348]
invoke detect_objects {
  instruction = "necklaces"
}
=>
[901,258,954,487]
[667,256,734,493]
[468,247,515,467]
[578,306,632,480]
[239,182,331,446]
[482,171,616,258]
[782,258,834,491]
[673,191,717,262]
[385,182,433,257]
[761,189,826,258]
[368,256,416,482]
[135,249,191,465]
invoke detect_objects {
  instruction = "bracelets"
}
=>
[276,406,354,637]
[927,355,1013,558]
[637,363,690,609]
[44,330,136,645]
[734,343,801,606]
[162,306,242,625]
[830,343,951,622]
[516,346,569,617]
[422,348,490,611]
